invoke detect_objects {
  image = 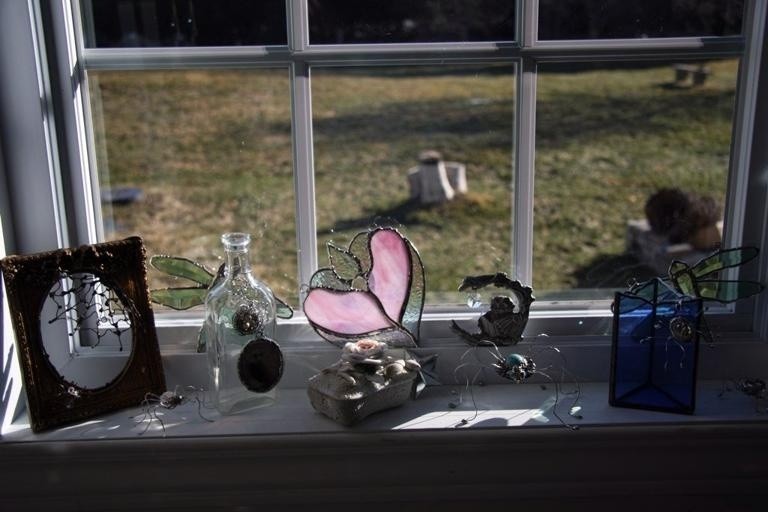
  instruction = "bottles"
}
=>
[205,231,278,416]
